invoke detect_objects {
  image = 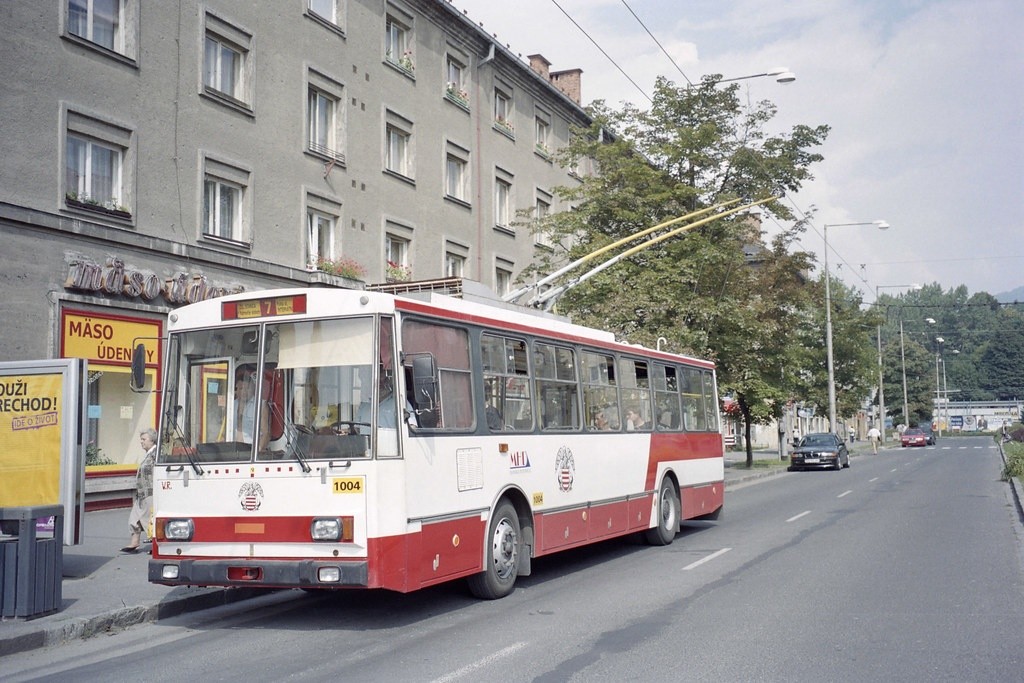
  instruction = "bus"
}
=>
[127,287,727,613]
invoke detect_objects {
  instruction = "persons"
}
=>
[218,371,272,458]
[483,379,503,431]
[344,362,417,455]
[593,410,612,431]
[865,424,882,455]
[644,406,670,428]
[791,425,802,448]
[626,404,650,429]
[117,426,158,556]
[848,425,857,444]
[1000,418,1014,447]
[977,415,989,430]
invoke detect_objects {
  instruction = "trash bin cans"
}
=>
[0,505,63,622]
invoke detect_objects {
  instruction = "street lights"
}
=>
[935,337,945,438]
[685,66,798,431]
[900,318,936,430]
[875,283,922,445]
[942,349,960,437]
[825,220,889,436]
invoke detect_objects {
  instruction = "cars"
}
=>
[920,428,937,447]
[791,433,851,472]
[901,429,927,448]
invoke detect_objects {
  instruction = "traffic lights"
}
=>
[1019,410,1024,424]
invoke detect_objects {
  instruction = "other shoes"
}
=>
[148,550,152,555]
[119,546,140,553]
